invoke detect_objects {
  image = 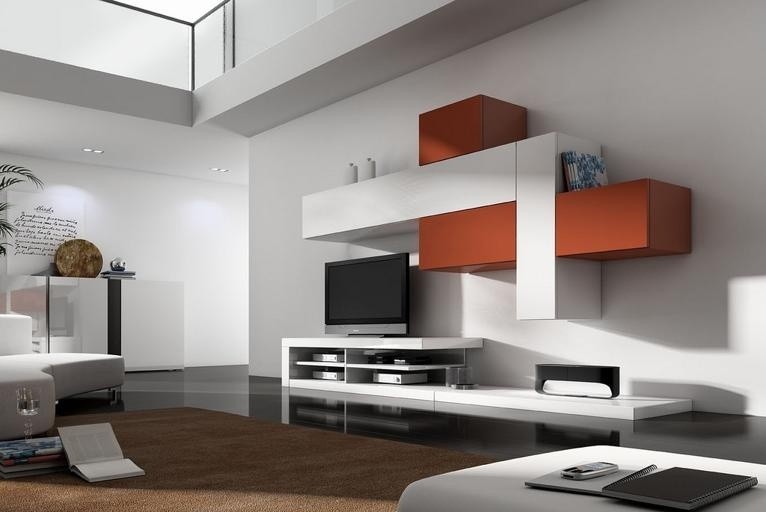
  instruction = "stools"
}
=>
[0,308,127,443]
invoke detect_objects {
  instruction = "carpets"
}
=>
[0,406,512,512]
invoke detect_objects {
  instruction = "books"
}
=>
[56,421,146,483]
[0,436,70,479]
[599,465,759,510]
[99,271,136,279]
[522,463,657,495]
[561,149,610,193]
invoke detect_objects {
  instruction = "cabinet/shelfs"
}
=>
[280,337,693,420]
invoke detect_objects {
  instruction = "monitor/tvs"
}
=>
[324,252,410,338]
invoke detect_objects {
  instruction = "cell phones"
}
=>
[560,461,619,480]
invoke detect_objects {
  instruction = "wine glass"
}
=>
[16,382,42,442]
